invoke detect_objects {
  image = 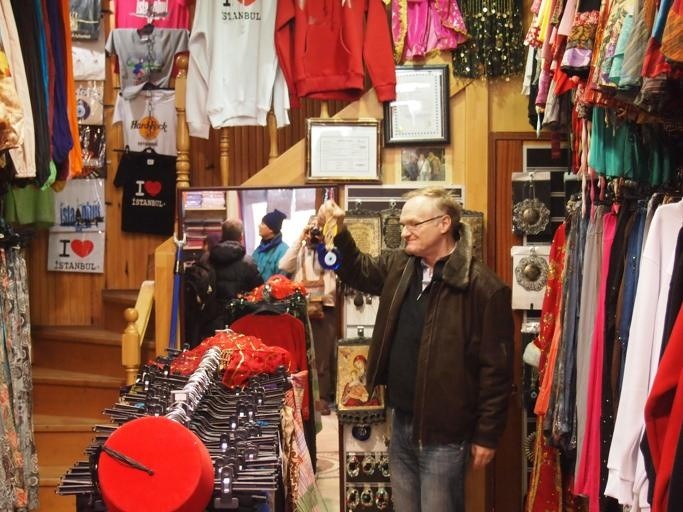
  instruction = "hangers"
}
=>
[0,223,37,248]
[571,168,683,207]
[113,148,178,160]
[119,83,175,96]
[137,14,155,38]
[54,343,292,512]
[225,281,306,317]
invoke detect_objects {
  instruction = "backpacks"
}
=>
[185,261,218,319]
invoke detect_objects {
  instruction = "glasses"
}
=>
[398,214,447,232]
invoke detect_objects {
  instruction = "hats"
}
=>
[262,210,287,235]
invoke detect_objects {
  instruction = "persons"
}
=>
[426,151,441,181]
[252,208,288,281]
[339,354,384,405]
[416,152,432,181]
[404,154,417,182]
[182,217,263,346]
[318,187,518,511]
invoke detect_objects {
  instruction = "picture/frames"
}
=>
[305,117,384,184]
[395,143,452,187]
[385,65,450,147]
[329,338,387,426]
